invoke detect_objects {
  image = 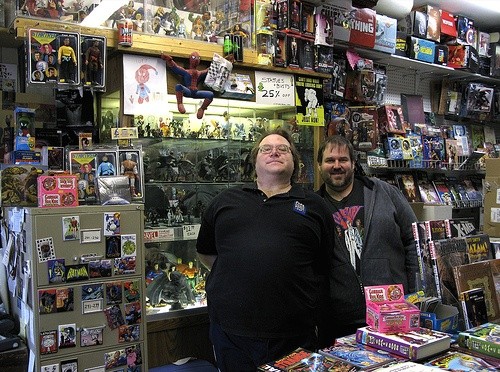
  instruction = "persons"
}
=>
[57,38,77,83]
[195,129,334,372]
[85,40,103,85]
[160,52,214,119]
[70,217,77,238]
[315,133,419,352]
[233,24,246,38]
[97,154,114,176]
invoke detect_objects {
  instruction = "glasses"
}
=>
[260,145,292,154]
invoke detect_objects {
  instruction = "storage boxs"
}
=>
[0,28,145,207]
[314,0,500,78]
[378,105,413,160]
[365,284,460,335]
[484,157,500,238]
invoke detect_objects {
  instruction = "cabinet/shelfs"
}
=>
[282,30,500,227]
[138,135,314,367]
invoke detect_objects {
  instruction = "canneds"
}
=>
[223,33,243,62]
[119,18,134,46]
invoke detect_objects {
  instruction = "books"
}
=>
[377,173,482,208]
[251,218,500,372]
